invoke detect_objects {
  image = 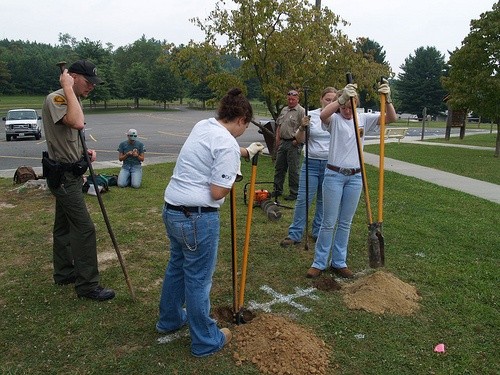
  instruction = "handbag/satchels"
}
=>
[45,160,62,188]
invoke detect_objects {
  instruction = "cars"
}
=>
[2,108,42,141]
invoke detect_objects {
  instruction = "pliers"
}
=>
[346,71,388,269]
[230,151,259,325]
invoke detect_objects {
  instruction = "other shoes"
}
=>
[271,192,282,197]
[306,268,321,278]
[77,285,115,302]
[219,327,232,345]
[280,236,301,246]
[330,265,354,278]
[285,195,295,201]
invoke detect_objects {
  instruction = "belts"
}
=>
[283,139,293,142]
[165,203,217,212]
[327,164,361,176]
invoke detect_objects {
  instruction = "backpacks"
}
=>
[83,171,117,196]
[14,165,37,184]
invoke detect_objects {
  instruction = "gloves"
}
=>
[338,83,358,105]
[235,161,243,182]
[377,78,393,104]
[245,142,264,162]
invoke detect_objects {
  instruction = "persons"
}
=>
[42,61,115,301]
[271,90,310,201]
[306,79,398,278]
[279,86,340,248]
[116,128,145,188]
[155,86,267,359]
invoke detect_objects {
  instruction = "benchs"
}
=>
[384,127,409,143]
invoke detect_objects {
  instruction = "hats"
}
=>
[127,129,137,141]
[68,61,104,85]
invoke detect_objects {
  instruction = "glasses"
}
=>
[287,92,298,96]
[128,134,137,137]
